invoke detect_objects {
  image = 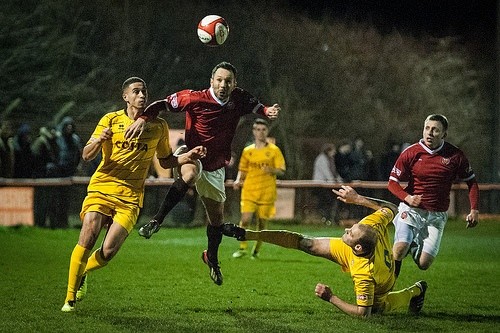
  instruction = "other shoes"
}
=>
[407,240,418,254]
[251,251,259,260]
[232,248,248,258]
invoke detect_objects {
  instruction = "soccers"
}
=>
[197,14,230,48]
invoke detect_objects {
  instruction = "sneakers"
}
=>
[410,281,427,313]
[202,250,223,285]
[221,223,247,241]
[76,275,87,300]
[61,301,76,312]
[138,219,161,239]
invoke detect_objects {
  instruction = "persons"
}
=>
[313,135,417,226]
[124,62,281,286]
[233,119,286,260]
[387,114,480,281]
[222,185,428,320]
[0,117,82,230]
[61,76,207,312]
